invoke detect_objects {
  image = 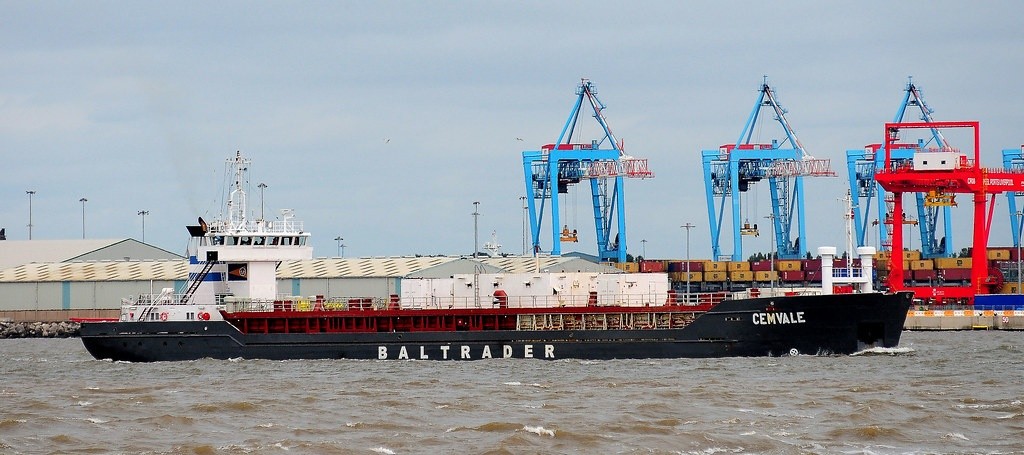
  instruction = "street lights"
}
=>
[25,191,36,240]
[763,213,774,297]
[519,196,529,254]
[137,210,149,242]
[470,202,480,256]
[79,198,87,240]
[258,183,267,219]
[641,239,647,259]
[680,223,695,306]
[1009,211,1024,294]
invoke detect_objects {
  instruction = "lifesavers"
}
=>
[161,312,167,320]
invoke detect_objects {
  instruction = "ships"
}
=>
[81,279,915,362]
